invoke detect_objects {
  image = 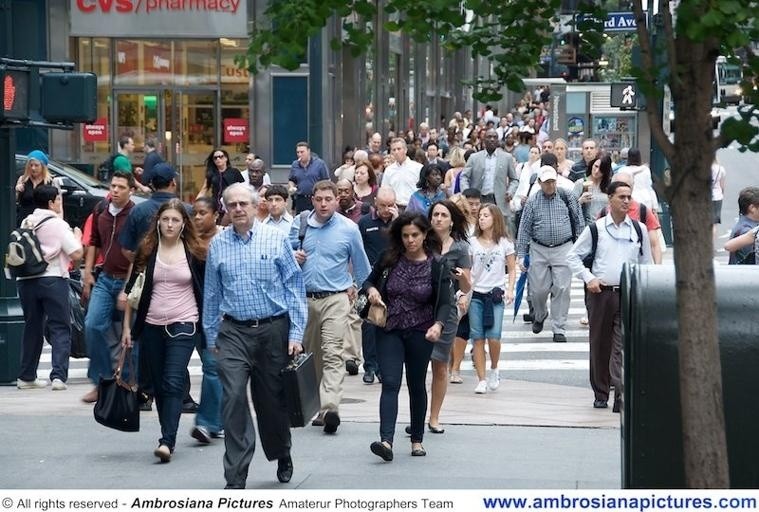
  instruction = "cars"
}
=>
[15,152,193,239]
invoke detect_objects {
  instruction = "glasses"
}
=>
[213,155,224,160]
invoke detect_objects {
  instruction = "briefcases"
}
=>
[279,352,322,428]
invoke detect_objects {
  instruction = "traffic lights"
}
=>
[609,82,638,109]
[1,65,38,122]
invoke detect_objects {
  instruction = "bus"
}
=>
[712,55,744,107]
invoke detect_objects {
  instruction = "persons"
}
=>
[13,85,758,489]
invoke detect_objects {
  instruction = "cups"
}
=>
[581,179,595,200]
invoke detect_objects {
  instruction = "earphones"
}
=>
[157,220,160,225]
[182,224,185,229]
[128,191,133,196]
[431,220,432,225]
[450,222,453,227]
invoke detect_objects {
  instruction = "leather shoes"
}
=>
[428,422,444,433]
[181,402,199,414]
[364,371,375,383]
[411,444,425,456]
[369,441,392,461]
[83,389,101,402]
[277,456,293,481]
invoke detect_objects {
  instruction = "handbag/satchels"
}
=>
[94,374,141,431]
[355,267,391,319]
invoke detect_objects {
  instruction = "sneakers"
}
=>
[345,360,358,375]
[554,333,566,343]
[17,379,48,388]
[449,375,464,384]
[322,409,341,434]
[153,450,170,463]
[488,371,500,391]
[311,413,324,426]
[532,311,549,333]
[474,378,488,394]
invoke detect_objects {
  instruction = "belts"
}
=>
[600,285,621,292]
[533,238,570,248]
[306,289,347,299]
[223,313,286,327]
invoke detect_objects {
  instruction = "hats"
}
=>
[535,165,557,183]
[25,149,50,168]
[151,162,178,186]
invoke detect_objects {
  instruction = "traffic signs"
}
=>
[572,10,648,32]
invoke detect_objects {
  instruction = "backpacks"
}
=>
[4,216,62,285]
[96,155,126,183]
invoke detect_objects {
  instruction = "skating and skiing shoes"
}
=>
[51,378,67,389]
